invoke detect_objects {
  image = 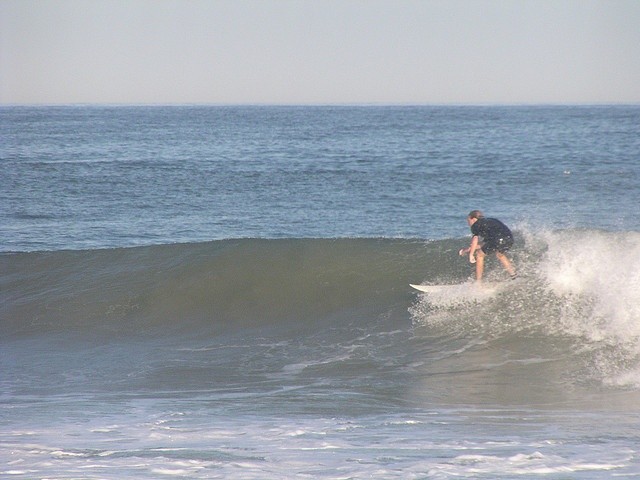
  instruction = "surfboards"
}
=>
[409,283,498,296]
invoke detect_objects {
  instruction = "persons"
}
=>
[458,210,517,290]
